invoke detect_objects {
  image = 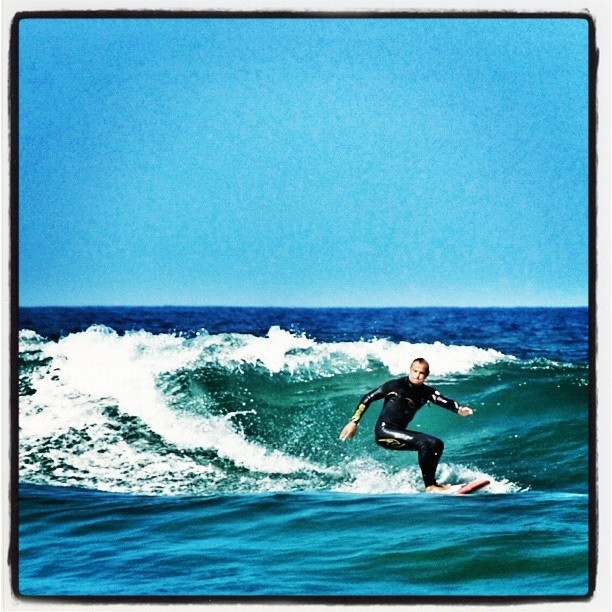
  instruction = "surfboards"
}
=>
[427,479,488,494]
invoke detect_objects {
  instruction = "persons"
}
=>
[339,357,474,494]
